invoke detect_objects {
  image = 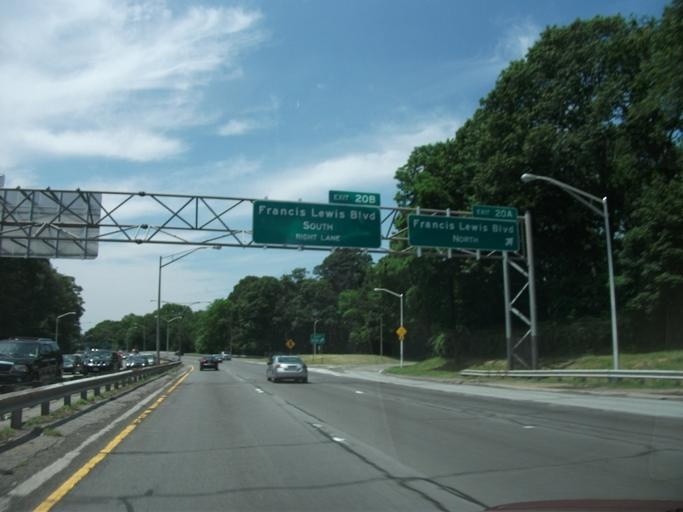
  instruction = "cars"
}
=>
[266,354,307,383]
[200,350,232,371]
[62,351,154,373]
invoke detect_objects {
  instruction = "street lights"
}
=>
[374,288,405,367]
[521,171,621,369]
[55,311,76,344]
[154,315,183,351]
[155,244,223,365]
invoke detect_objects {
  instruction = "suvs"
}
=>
[0,337,63,394]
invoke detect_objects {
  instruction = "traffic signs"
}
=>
[406,203,519,252]
[309,334,325,345]
[251,191,380,248]
